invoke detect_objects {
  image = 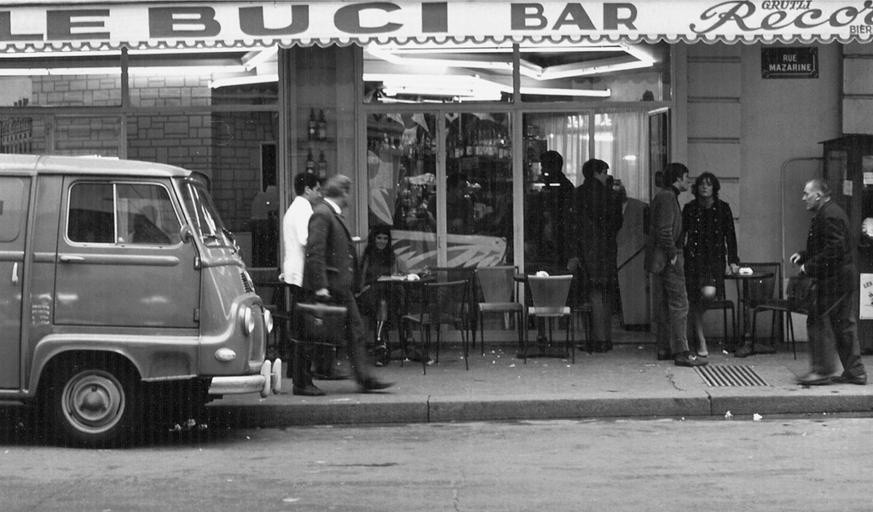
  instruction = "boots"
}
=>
[373,320,394,366]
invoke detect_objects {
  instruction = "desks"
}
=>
[722,271,781,359]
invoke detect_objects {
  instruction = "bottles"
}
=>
[368,125,512,163]
[305,151,326,180]
[306,107,327,141]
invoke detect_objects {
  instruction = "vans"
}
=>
[0,149,286,451]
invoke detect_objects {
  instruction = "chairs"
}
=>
[701,279,737,347]
[751,274,801,362]
[737,259,784,345]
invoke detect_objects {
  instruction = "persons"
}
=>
[291,175,393,395]
[524,151,570,270]
[280,174,350,380]
[678,173,739,356]
[359,225,436,366]
[788,177,866,386]
[563,160,625,354]
[610,180,651,251]
[424,175,477,233]
[649,163,711,366]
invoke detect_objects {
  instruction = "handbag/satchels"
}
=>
[788,269,819,316]
[291,294,348,347]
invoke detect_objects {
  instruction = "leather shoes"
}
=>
[793,369,833,386]
[831,369,870,386]
[579,339,614,353]
[292,381,324,396]
[357,380,396,392]
[660,348,709,366]
